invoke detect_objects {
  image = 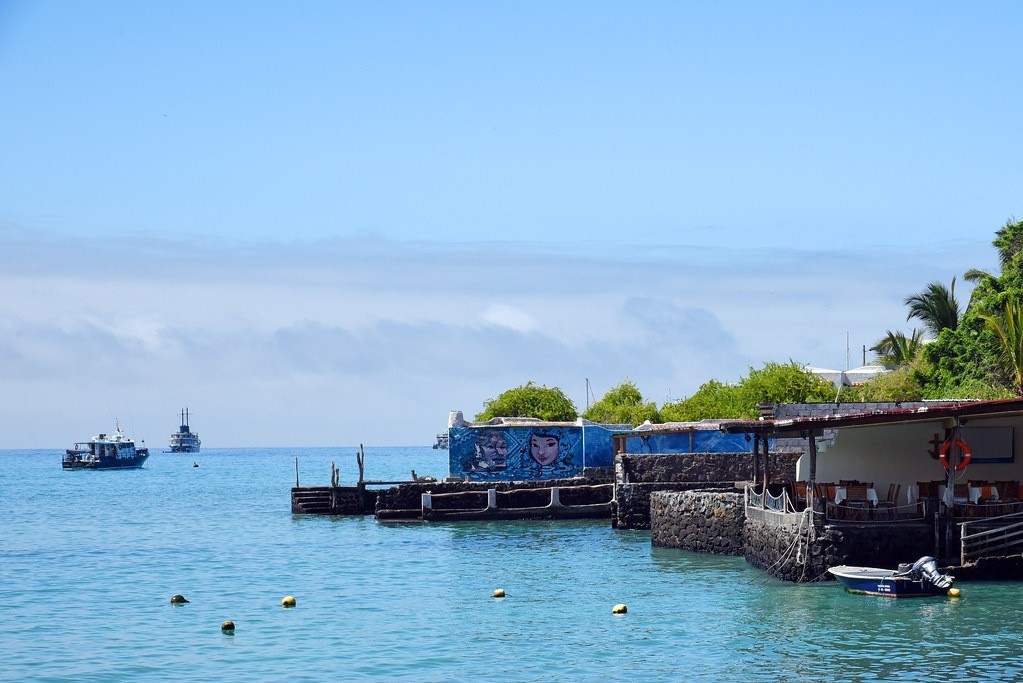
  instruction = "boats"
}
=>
[162,408,202,452]
[828,557,956,598]
[62,417,150,471]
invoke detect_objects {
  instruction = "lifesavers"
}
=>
[938,436,970,470]
[76,445,79,450]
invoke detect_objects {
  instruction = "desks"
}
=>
[954,486,999,505]
[834,488,878,507]
[906,485,946,505]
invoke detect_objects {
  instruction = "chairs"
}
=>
[776,478,1022,519]
